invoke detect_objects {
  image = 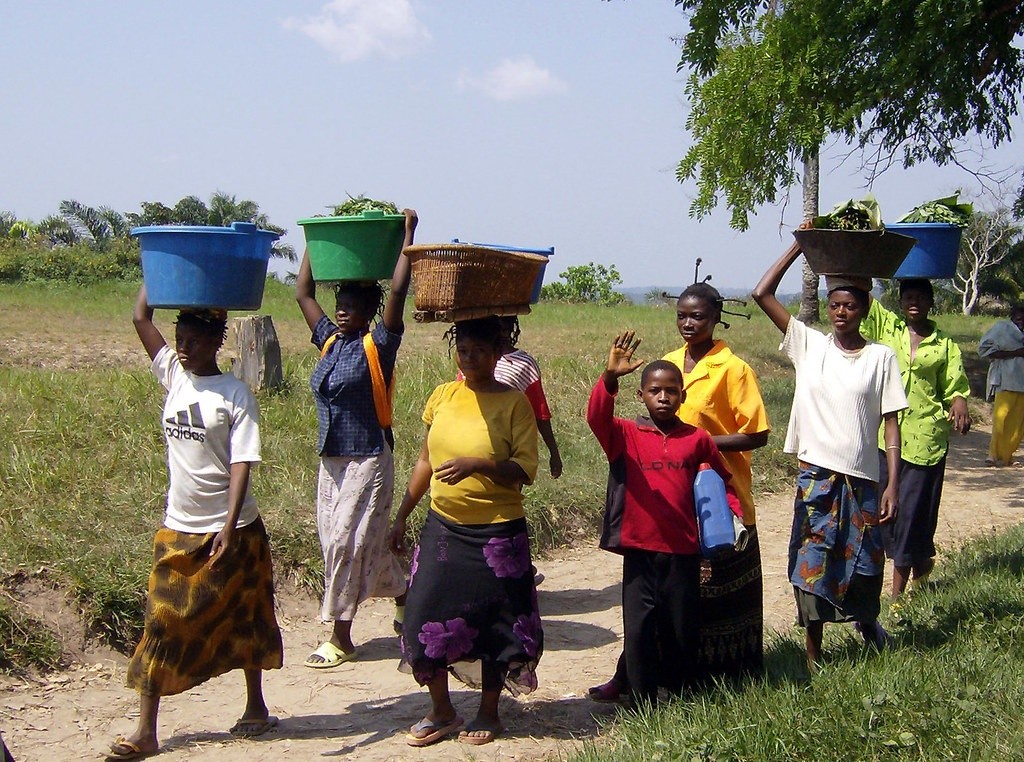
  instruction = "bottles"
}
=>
[693,462,734,547]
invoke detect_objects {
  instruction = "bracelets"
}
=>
[884,446,901,455]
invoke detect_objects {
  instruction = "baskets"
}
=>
[403,244,550,311]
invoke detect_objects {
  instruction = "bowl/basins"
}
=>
[133,220,279,312]
[792,228,916,280]
[450,237,555,302]
[299,211,417,283]
[882,222,968,278]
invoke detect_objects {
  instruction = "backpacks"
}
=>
[322,329,397,452]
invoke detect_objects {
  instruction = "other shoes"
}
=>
[589,679,623,703]
[907,559,935,597]
[850,620,894,654]
[888,603,903,622]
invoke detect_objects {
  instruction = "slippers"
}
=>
[406,715,464,745]
[99,736,161,759]
[458,720,503,745]
[393,603,406,635]
[1010,461,1024,470]
[534,573,544,586]
[232,716,278,736]
[985,453,995,466]
[304,641,364,667]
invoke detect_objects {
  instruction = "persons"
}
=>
[389,314,562,746]
[861,279,971,617]
[296,209,418,668]
[586,283,772,712]
[977,301,1024,467]
[101,279,284,759]
[752,220,910,680]
